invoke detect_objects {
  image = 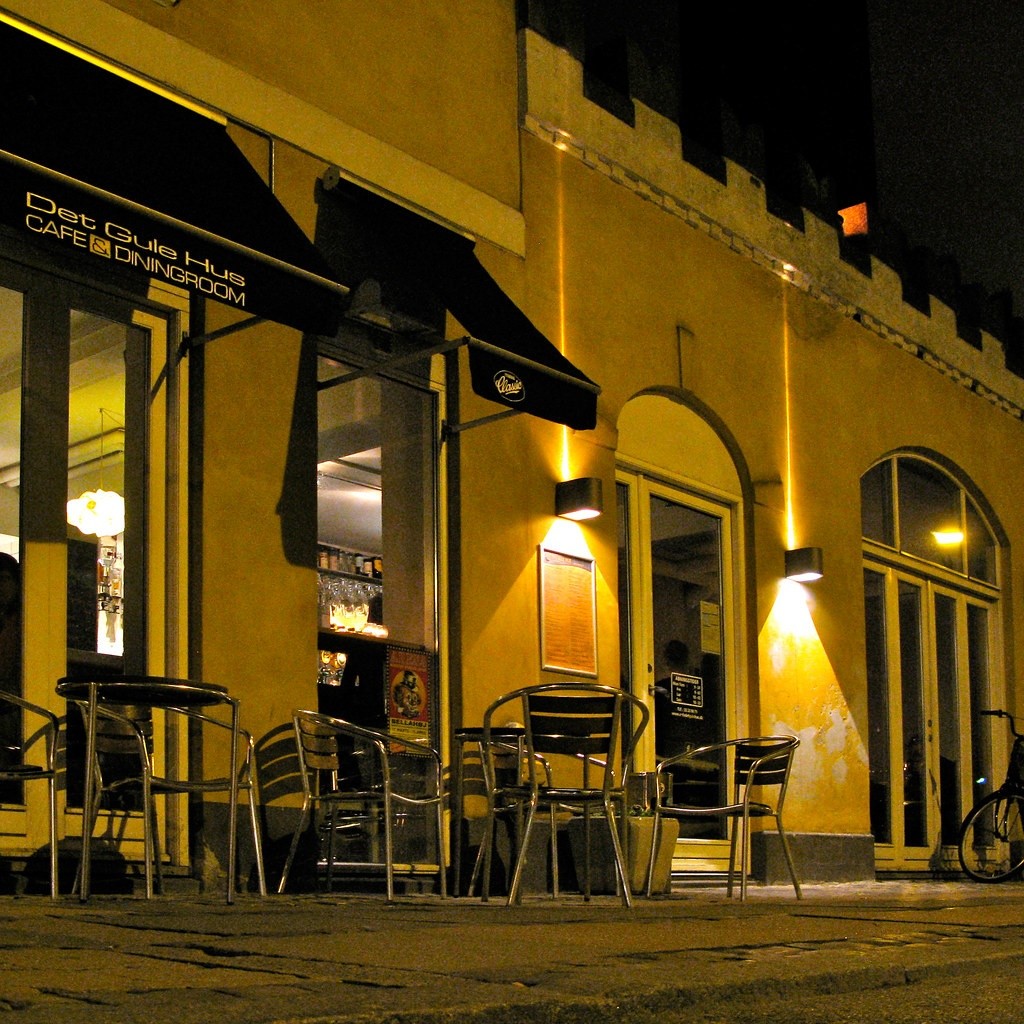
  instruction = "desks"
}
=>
[56,676,240,905]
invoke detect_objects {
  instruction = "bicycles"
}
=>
[957,709,1024,882]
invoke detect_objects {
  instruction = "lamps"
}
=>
[555,477,603,521]
[67,407,126,537]
[784,548,824,582]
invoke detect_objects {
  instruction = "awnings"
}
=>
[326,171,602,428]
[0,22,350,335]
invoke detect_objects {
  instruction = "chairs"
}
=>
[276,709,447,904]
[643,734,803,902]
[0,690,58,900]
[455,727,559,901]
[481,681,650,907]
[70,699,268,898]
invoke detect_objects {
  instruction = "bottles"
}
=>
[318,549,383,581]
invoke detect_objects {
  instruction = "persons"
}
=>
[655,641,713,754]
[338,596,381,783]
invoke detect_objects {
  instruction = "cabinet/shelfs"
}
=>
[316,541,424,654]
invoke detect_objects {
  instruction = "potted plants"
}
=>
[566,804,680,895]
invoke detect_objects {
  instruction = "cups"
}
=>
[317,573,383,634]
